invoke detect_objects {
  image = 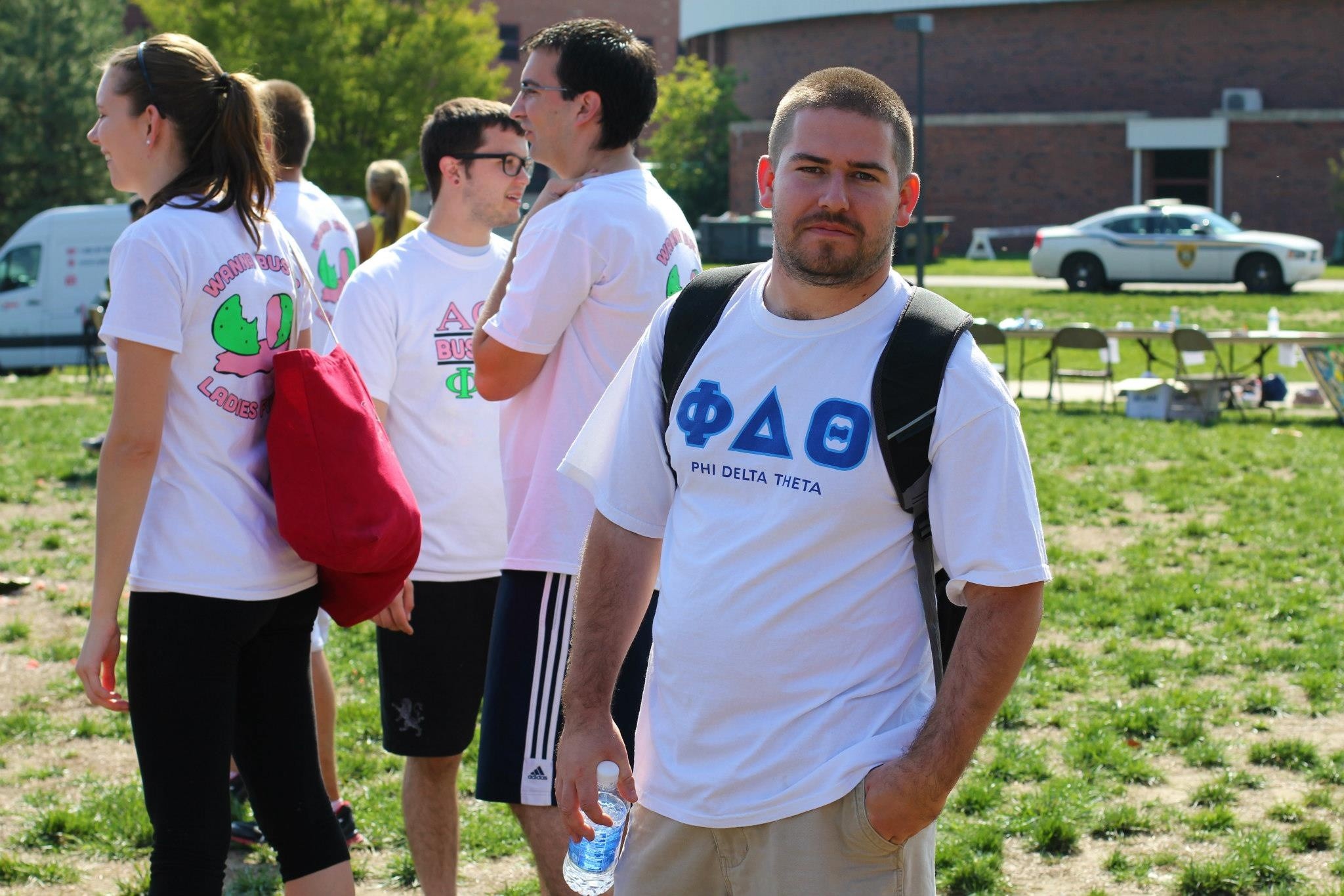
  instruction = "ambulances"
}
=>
[0,203,148,373]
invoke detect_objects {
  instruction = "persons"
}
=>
[198,68,359,847]
[336,90,532,896]
[555,68,1051,896]
[479,18,701,894]
[355,156,426,263]
[77,31,353,896]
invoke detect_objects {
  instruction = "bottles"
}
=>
[1170,306,1179,333]
[1267,307,1280,337]
[563,760,631,896]
[1154,321,1177,333]
[999,308,1043,331]
[1116,321,1134,332]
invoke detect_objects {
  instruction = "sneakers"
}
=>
[231,800,365,848]
[230,773,249,804]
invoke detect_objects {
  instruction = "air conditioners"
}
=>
[1222,88,1263,112]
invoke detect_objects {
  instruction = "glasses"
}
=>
[521,82,577,101]
[455,152,534,179]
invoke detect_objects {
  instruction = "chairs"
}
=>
[970,318,1009,385]
[1171,326,1247,422]
[1049,324,1118,416]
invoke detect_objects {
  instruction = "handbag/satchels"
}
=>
[265,238,422,627]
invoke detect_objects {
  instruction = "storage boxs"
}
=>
[1112,377,1201,421]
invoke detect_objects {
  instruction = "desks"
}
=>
[1002,328,1344,420]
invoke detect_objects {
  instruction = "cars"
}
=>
[1024,203,1331,297]
